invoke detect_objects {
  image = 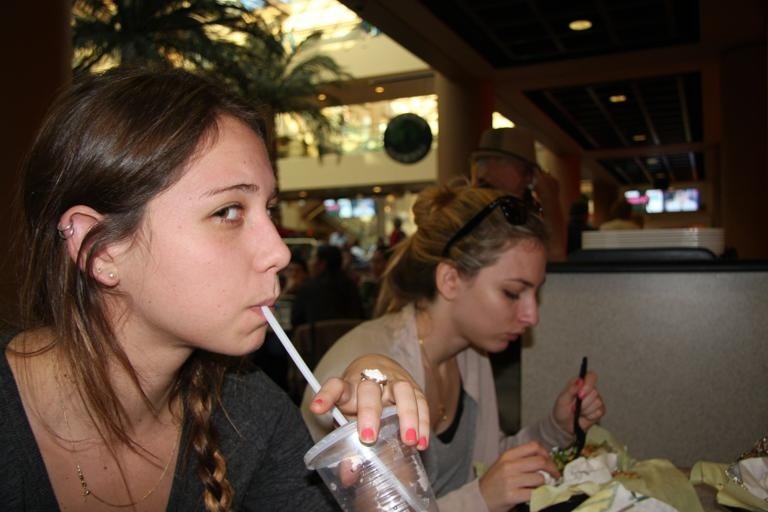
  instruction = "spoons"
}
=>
[562,356,588,466]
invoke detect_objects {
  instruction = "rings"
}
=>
[360,368,389,393]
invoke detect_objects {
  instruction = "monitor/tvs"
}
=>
[663,184,700,214]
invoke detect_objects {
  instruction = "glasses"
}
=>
[441,194,544,256]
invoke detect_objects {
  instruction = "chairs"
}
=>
[285,318,359,406]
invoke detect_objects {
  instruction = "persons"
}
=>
[0,66,431,512]
[299,173,607,512]
[277,217,406,328]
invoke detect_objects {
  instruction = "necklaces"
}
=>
[53,345,182,508]
[418,331,449,421]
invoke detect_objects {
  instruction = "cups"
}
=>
[302,405,442,512]
